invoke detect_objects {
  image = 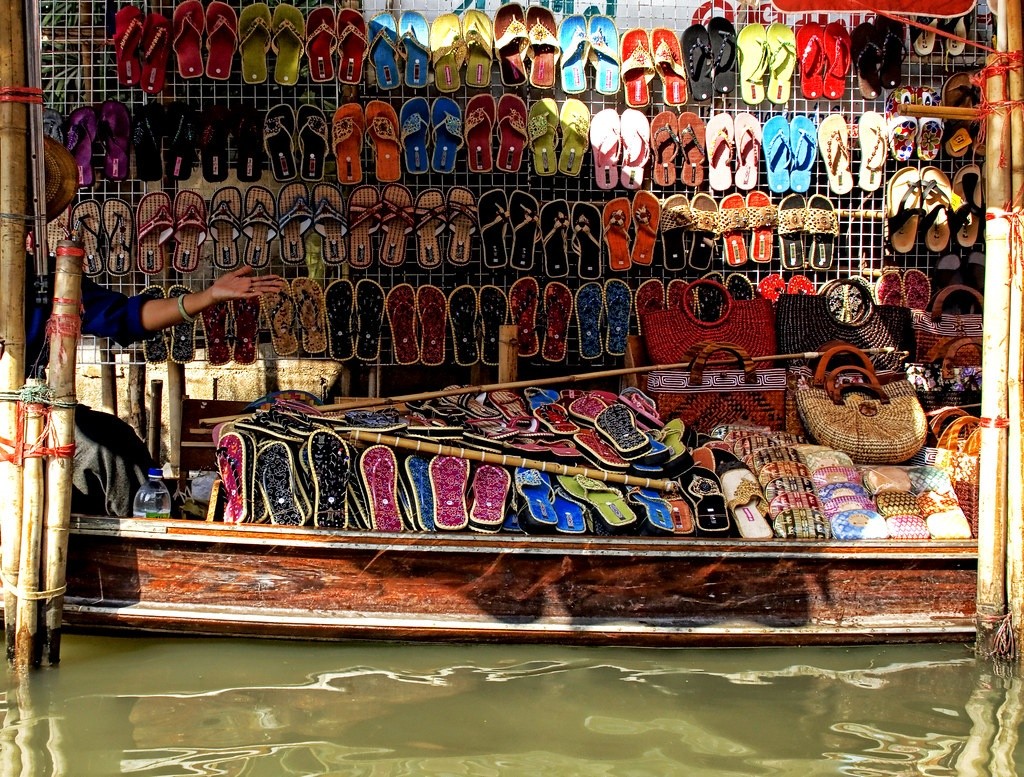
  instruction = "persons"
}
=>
[23,127,286,516]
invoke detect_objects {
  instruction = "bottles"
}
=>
[133,467,171,518]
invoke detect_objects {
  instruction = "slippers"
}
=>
[212,384,773,539]
[26,0,986,366]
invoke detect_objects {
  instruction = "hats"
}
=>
[26,130,80,232]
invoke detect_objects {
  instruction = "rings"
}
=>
[252,286,256,292]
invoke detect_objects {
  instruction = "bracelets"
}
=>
[178,294,196,323]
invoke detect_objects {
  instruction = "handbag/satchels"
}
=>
[638,279,983,539]
